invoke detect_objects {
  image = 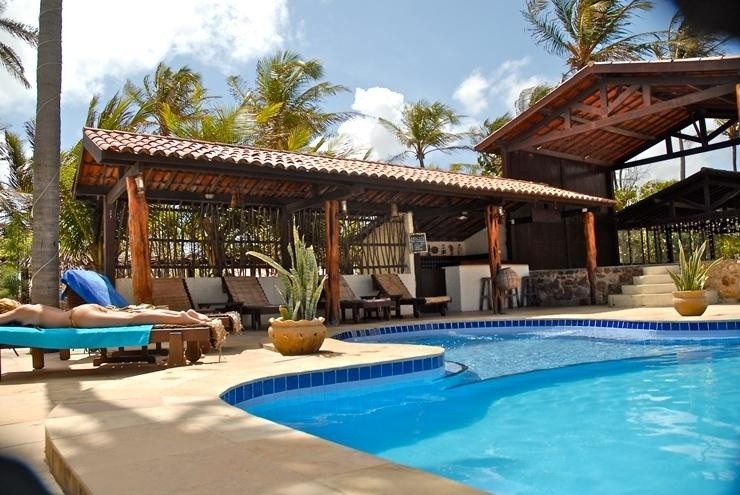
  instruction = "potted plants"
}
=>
[241,214,327,357]
[665,230,724,316]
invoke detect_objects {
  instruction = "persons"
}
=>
[0,298,212,328]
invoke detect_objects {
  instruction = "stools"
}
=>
[478,278,515,311]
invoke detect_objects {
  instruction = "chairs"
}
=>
[0,322,211,366]
[314,272,391,323]
[149,277,239,333]
[219,275,286,328]
[372,272,450,318]
[61,268,232,352]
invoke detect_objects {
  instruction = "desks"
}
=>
[199,302,243,315]
[361,294,403,320]
